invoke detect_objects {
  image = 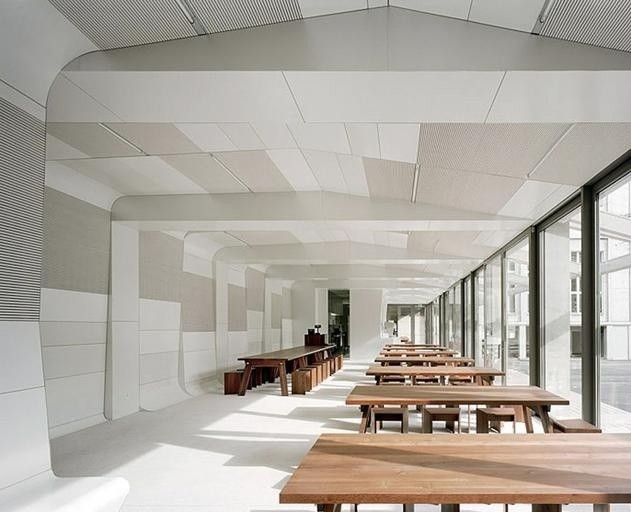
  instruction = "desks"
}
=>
[345,385,569,434]
[236,343,337,396]
[280,433,629,512]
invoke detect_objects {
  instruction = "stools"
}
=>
[421,407,461,433]
[552,418,602,433]
[365,343,507,410]
[291,353,342,395]
[370,407,409,434]
[224,366,268,395]
[476,408,515,433]
